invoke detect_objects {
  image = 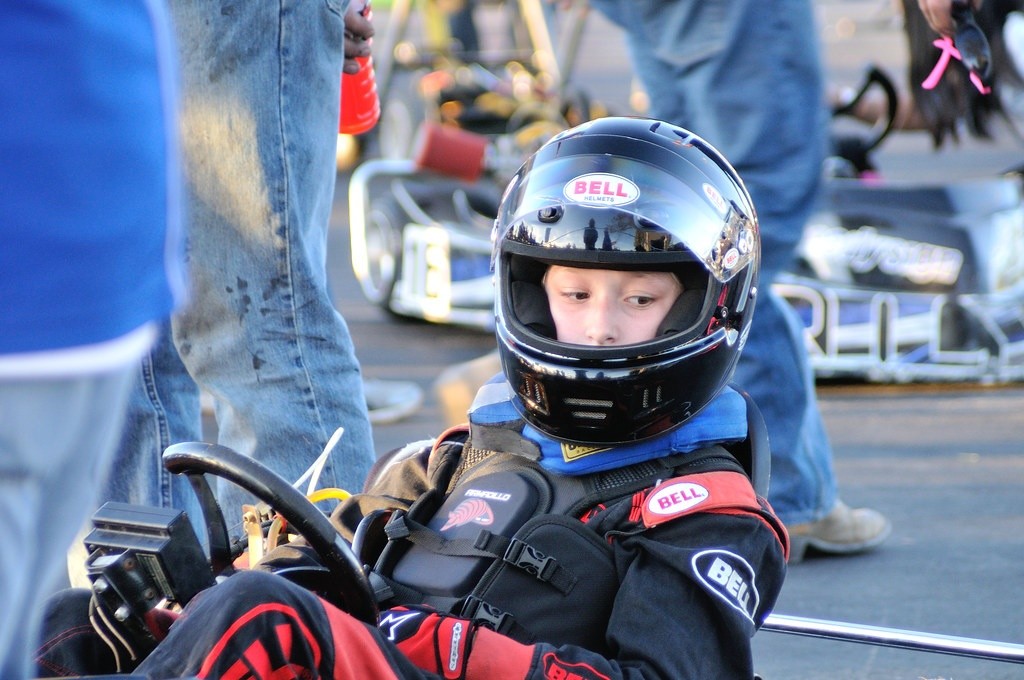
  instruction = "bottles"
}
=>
[337,0,382,135]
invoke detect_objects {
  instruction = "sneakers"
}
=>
[786,500,892,566]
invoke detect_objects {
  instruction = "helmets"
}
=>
[491,115,763,450]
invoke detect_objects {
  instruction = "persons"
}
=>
[31,118,791,680]
[167,0,986,586]
[0,1,211,680]
